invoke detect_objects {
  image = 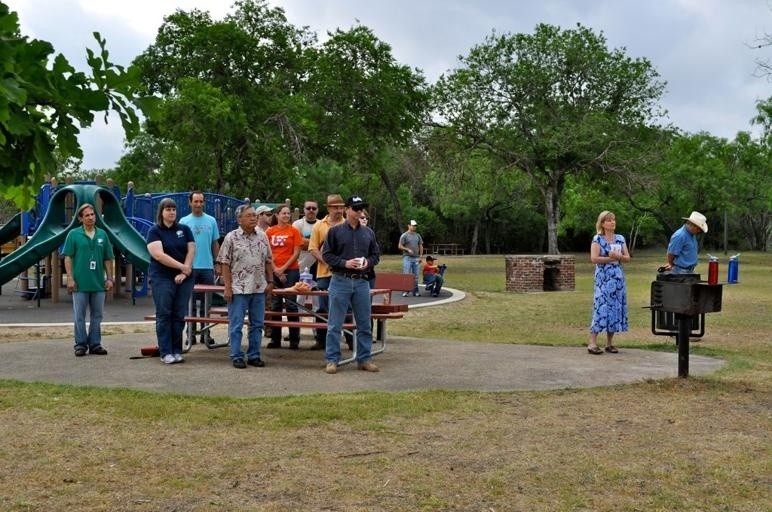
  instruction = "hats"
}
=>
[426,256,436,261]
[683,212,711,232]
[325,193,346,207]
[407,219,418,226]
[346,195,369,208]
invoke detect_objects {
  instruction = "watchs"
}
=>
[266,279,276,285]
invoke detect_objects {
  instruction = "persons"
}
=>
[60,203,115,356]
[217,204,274,369]
[399,220,424,297]
[147,198,195,364]
[664,212,708,341]
[423,255,446,296]
[588,211,630,354]
[323,196,380,374]
[358,209,376,343]
[309,193,353,353]
[254,205,274,338]
[266,206,302,350]
[179,192,223,345]
[284,200,323,341]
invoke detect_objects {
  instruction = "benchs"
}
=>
[282,273,416,340]
[208,309,404,355]
[145,313,355,367]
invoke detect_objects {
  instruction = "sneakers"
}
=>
[426,284,433,290]
[326,363,338,374]
[413,292,421,296]
[357,361,379,371]
[175,354,185,363]
[403,292,408,296]
[433,293,438,296]
[161,354,174,363]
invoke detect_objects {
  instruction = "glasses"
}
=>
[305,207,318,211]
[262,212,274,217]
[350,206,365,212]
[360,217,370,220]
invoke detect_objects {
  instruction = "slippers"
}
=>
[586,345,602,355]
[606,345,619,354]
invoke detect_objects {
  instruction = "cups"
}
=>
[355,257,365,268]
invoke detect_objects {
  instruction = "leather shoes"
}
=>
[267,342,281,348]
[186,337,196,346]
[289,343,298,349]
[89,347,108,355]
[247,359,266,367]
[199,336,215,344]
[75,349,88,356]
[311,342,327,350]
[232,359,245,368]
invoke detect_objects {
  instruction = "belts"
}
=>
[334,271,370,279]
[679,266,697,272]
